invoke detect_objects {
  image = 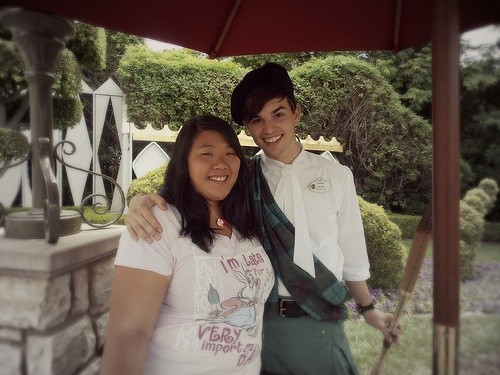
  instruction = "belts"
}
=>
[277,298,311,318]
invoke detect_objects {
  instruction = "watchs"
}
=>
[356,301,375,314]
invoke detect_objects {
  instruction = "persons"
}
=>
[100,113,276,375]
[126,63,403,375]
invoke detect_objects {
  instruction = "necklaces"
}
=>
[208,206,228,228]
